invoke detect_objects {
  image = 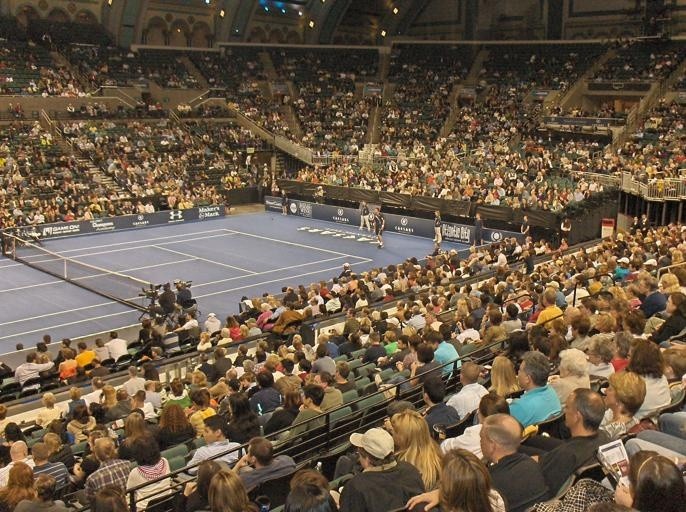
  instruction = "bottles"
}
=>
[313,461,322,472]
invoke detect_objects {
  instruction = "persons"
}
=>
[0,0,686,512]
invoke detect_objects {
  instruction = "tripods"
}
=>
[138,299,157,321]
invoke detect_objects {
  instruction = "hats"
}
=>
[617,257,629,264]
[349,427,396,460]
[643,259,657,266]
[546,281,559,289]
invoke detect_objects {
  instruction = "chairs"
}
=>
[1,48,686,510]
